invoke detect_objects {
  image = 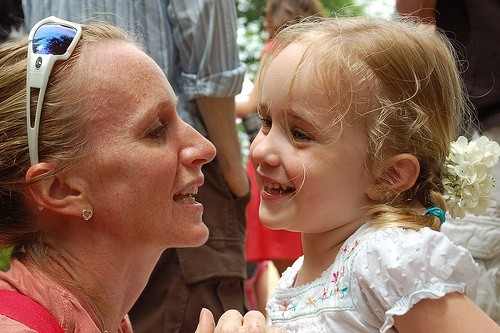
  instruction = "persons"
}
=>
[250,16,500,333]
[0,22,291,333]
[397,0,500,146]
[21,0,250,333]
[235,0,341,318]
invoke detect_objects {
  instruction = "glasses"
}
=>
[26,15,87,210]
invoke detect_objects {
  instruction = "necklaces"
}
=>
[37,244,119,332]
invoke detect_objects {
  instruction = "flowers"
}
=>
[440,135,500,220]
